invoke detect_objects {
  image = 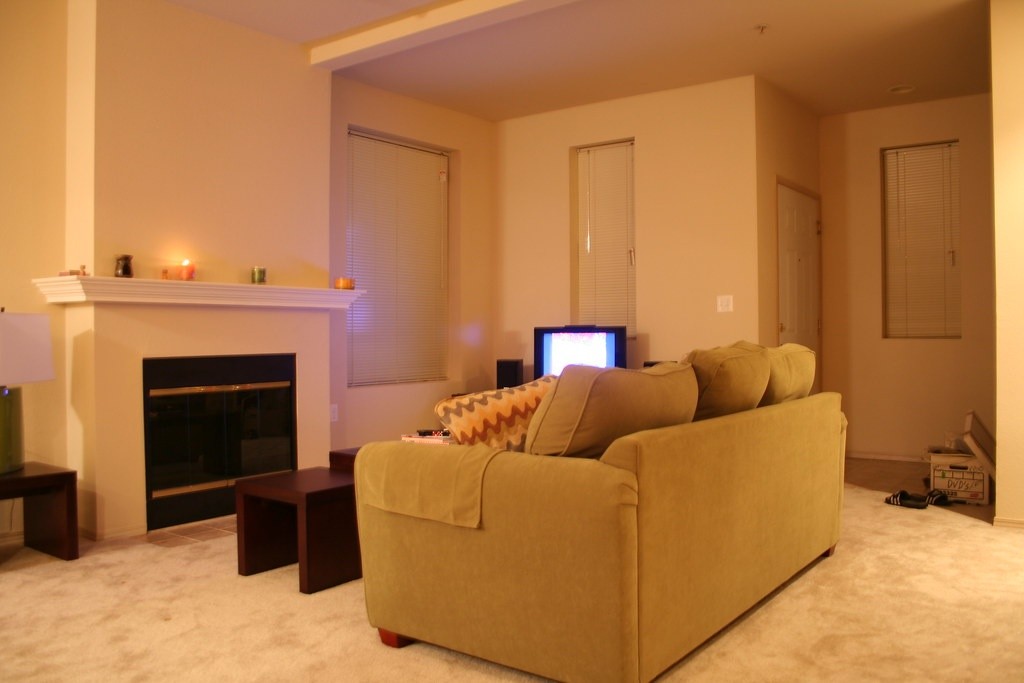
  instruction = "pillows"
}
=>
[434,374,557,453]
[524,363,700,459]
[682,340,771,422]
[761,343,816,406]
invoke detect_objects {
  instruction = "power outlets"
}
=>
[330,403,338,422]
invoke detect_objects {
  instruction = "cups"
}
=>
[335,278,355,289]
[251,266,266,284]
[175,265,195,279]
[115,254,134,278]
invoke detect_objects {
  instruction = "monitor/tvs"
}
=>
[534,326,626,380]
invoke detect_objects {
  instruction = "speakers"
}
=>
[496,359,523,389]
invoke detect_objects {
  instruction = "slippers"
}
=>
[884,490,928,509]
[910,488,953,507]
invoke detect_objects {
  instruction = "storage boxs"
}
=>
[927,408,996,507]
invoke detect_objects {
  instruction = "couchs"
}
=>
[354,391,848,683]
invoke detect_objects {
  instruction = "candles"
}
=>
[334,275,356,290]
[178,259,196,282]
[251,263,269,285]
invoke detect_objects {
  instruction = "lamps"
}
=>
[0,312,55,475]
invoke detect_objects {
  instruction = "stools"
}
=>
[235,466,363,595]
[0,461,79,562]
